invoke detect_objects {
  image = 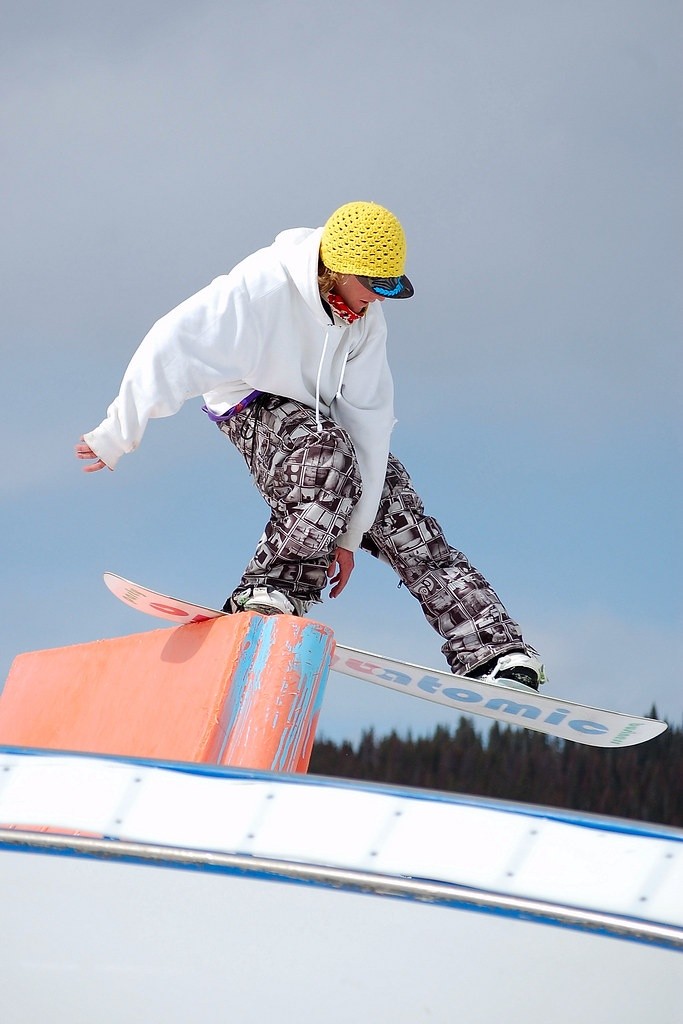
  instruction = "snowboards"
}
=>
[102,570,669,748]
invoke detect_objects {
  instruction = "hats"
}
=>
[320,200,414,299]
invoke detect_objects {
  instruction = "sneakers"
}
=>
[220,584,295,615]
[472,648,547,694]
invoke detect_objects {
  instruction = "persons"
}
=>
[76,201,546,693]
